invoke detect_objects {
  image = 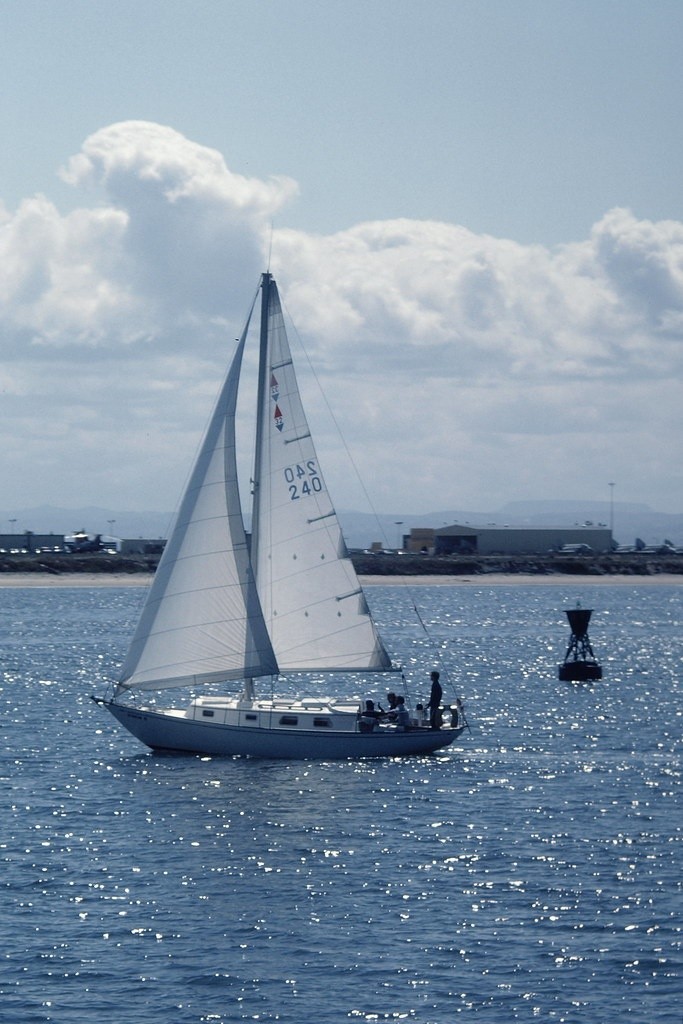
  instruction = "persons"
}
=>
[361,700,384,719]
[424,671,442,731]
[386,692,406,720]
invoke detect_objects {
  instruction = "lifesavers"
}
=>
[438,705,459,728]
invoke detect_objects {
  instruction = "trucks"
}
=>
[62,534,104,554]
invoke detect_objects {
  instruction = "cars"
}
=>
[557,542,594,557]
[359,550,418,555]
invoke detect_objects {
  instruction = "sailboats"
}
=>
[87,270,471,763]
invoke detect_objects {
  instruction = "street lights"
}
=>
[8,519,17,535]
[608,482,616,554]
[107,520,116,537]
